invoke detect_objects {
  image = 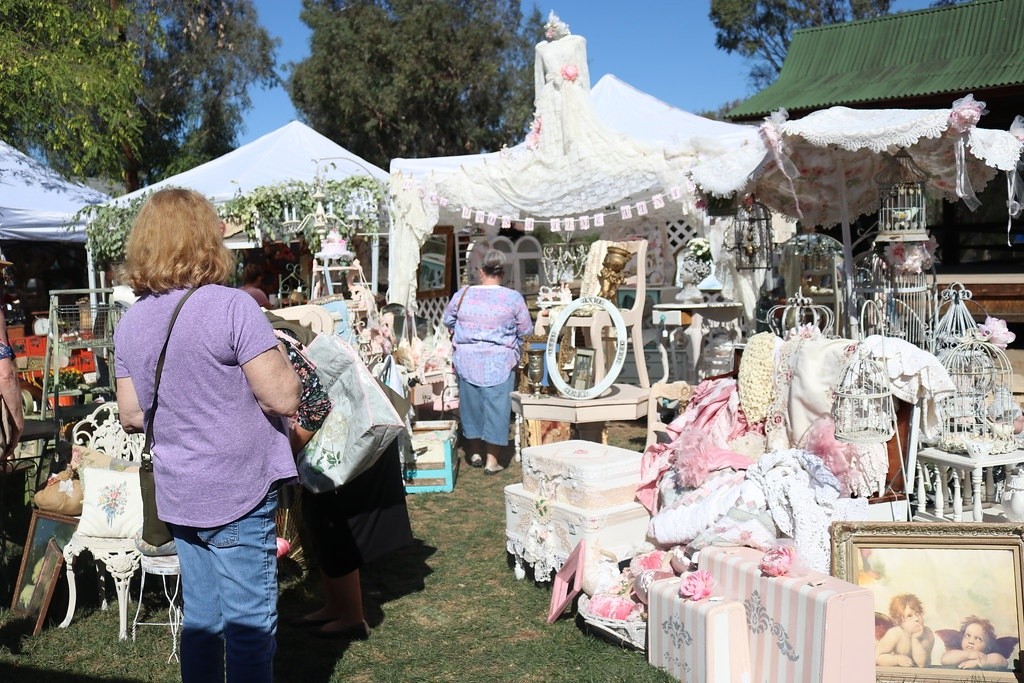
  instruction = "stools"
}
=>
[132,554,184,665]
[57,467,144,642]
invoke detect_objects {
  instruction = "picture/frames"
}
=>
[11,509,81,613]
[27,540,65,635]
[830,522,1024,683]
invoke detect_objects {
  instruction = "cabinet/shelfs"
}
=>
[37,288,121,469]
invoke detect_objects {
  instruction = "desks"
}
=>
[654,302,745,379]
[510,383,650,463]
[919,448,1024,523]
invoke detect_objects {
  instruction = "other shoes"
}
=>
[469,454,483,467]
[308,621,370,637]
[287,612,336,627]
[485,465,504,475]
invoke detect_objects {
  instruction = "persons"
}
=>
[0,307,24,472]
[442,249,535,476]
[265,311,414,640]
[113,188,302,683]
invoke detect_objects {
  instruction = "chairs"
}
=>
[534,238,650,389]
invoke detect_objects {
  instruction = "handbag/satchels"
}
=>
[139,463,174,547]
[273,329,405,494]
[398,313,422,371]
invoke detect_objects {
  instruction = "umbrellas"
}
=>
[684,94,1024,340]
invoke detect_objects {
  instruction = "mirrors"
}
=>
[546,296,628,400]
[415,224,454,299]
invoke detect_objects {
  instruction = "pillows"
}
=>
[78,467,143,538]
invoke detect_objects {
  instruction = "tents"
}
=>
[0,137,113,323]
[389,75,797,314]
[89,119,390,263]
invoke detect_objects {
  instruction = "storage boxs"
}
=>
[398,419,459,493]
